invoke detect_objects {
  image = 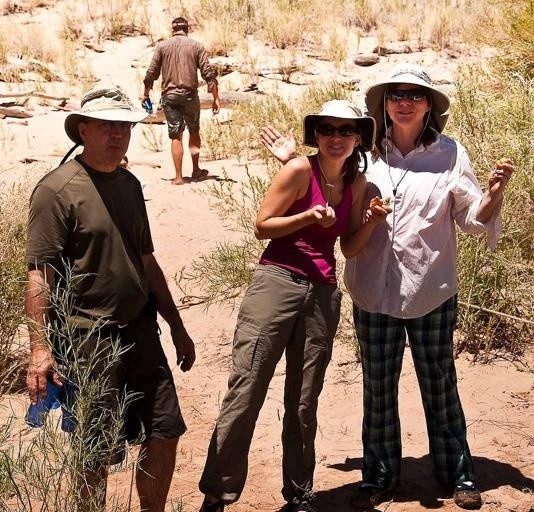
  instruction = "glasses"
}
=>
[386,87,425,102]
[315,123,361,138]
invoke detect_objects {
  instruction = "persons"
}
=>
[260,64,515,511]
[24,84,196,512]
[141,17,221,184]
[199,98,393,512]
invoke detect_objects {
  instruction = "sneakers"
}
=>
[453,480,482,509]
[348,483,387,508]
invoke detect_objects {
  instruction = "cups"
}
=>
[25,376,64,428]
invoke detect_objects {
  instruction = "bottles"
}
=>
[143,99,152,114]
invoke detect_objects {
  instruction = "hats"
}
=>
[64,86,150,147]
[300,99,377,152]
[364,63,450,134]
